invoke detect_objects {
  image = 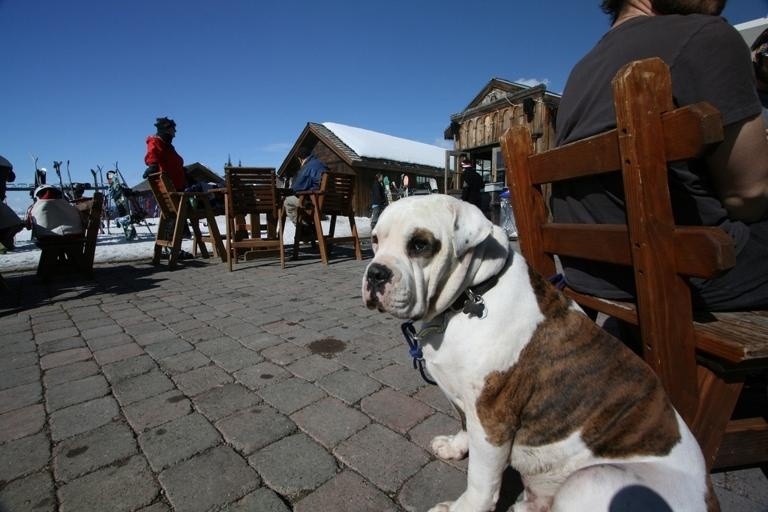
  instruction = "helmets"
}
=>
[460,160,472,168]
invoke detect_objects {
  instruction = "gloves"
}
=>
[143,164,157,179]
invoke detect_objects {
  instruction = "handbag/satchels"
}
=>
[185,175,256,209]
[295,224,316,242]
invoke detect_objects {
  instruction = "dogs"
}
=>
[362,194,721,512]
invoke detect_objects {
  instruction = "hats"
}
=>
[154,118,176,129]
[0,156,15,182]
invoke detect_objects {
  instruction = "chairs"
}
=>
[36,193,104,274]
[146,165,363,272]
[499,57,768,469]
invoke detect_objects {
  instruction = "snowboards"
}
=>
[383,176,392,204]
[429,178,438,194]
[34,168,46,187]
[403,176,409,197]
[110,177,136,241]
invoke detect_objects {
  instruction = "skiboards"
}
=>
[54,160,65,199]
[91,165,111,234]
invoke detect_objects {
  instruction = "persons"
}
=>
[145,115,194,262]
[547,1,768,417]
[283,147,336,243]
[368,172,386,233]
[459,160,485,208]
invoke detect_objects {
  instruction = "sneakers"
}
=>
[162,250,193,259]
